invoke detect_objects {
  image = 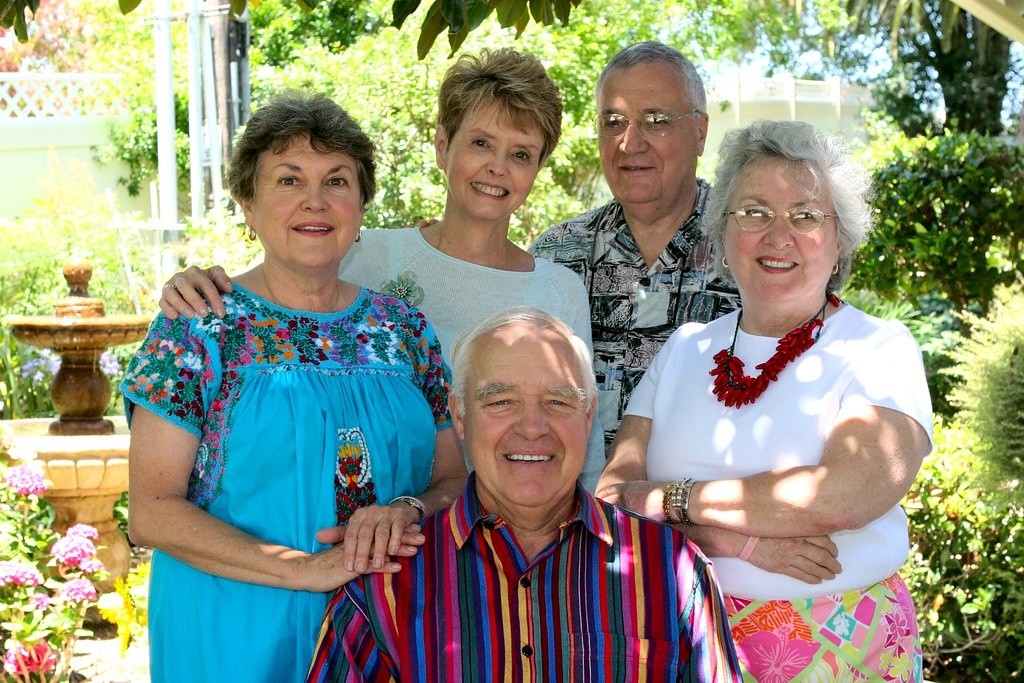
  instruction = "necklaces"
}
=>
[709,294,840,408]
[262,270,339,314]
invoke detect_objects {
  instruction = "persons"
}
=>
[120,41,933,683]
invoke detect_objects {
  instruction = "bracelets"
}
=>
[738,536,759,560]
[672,476,697,526]
[388,495,427,519]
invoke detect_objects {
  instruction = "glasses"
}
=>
[724,204,838,234]
[594,113,696,137]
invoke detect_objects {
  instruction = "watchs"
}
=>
[663,481,680,524]
